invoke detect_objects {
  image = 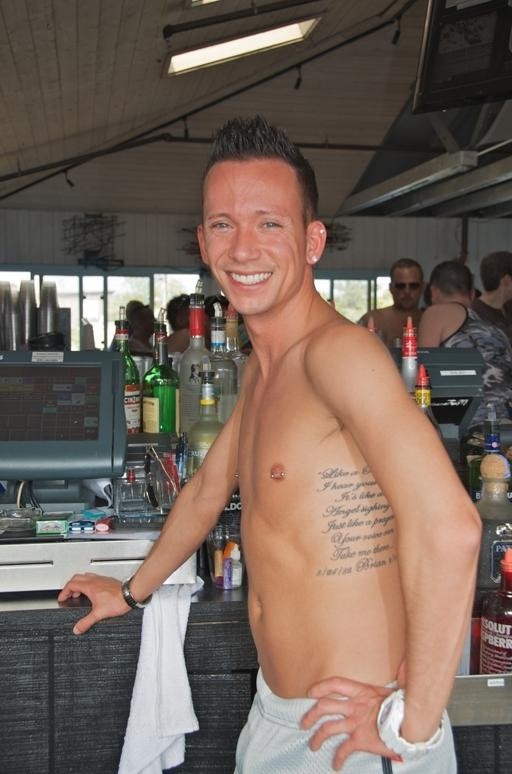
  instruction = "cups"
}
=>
[0,282,18,352]
[40,283,57,333]
[19,279,37,349]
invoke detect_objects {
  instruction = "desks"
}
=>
[0,586,259,773]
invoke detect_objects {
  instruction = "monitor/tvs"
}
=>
[0,349,128,514]
[389,346,487,458]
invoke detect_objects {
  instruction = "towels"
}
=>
[113,583,202,774]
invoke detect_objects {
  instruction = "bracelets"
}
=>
[122,580,154,612]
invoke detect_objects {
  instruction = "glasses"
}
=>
[391,282,420,291]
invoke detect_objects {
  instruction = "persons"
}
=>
[417,260,511,435]
[471,251,510,339]
[358,259,427,348]
[56,113,485,774]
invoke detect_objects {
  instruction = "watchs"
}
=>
[376,688,445,765]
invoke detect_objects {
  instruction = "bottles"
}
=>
[224,302,250,388]
[414,365,445,447]
[111,320,141,435]
[476,420,512,503]
[210,315,238,425]
[468,455,510,672]
[400,316,419,397]
[142,322,176,433]
[184,370,225,479]
[477,549,512,675]
[179,292,212,436]
